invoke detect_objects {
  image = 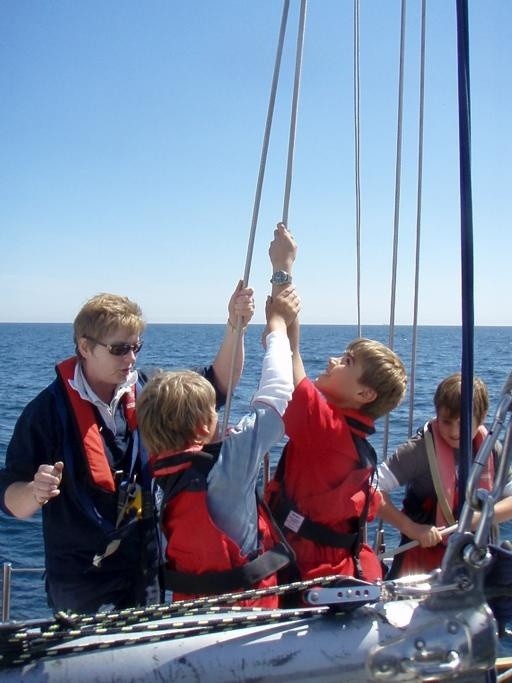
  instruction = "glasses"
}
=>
[83,336,144,356]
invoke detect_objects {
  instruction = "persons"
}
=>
[263,221,405,607]
[367,372,511,581]
[0,278,259,617]
[134,284,308,610]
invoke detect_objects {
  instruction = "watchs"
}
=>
[271,269,291,284]
[35,496,51,506]
